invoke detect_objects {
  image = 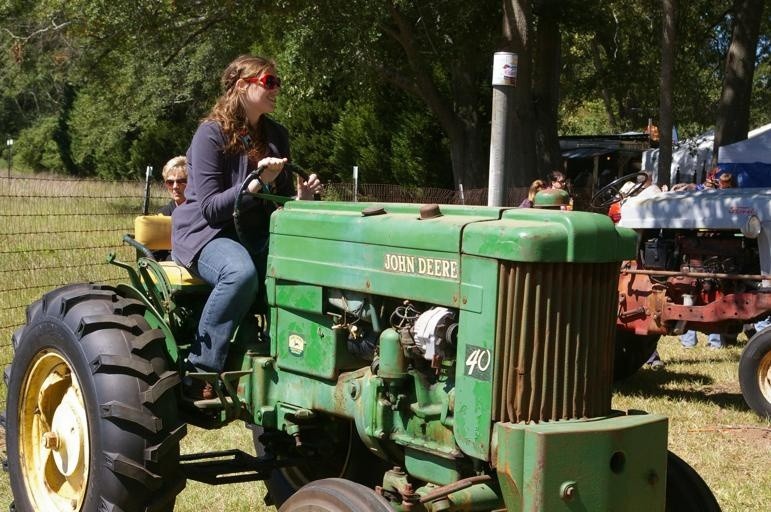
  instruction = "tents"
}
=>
[641,123,771,187]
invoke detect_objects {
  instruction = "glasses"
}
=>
[555,180,565,183]
[165,178,187,186]
[243,75,281,90]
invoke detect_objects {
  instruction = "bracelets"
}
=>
[254,176,265,188]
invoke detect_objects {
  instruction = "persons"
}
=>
[678,179,726,349]
[146,155,189,260]
[565,179,574,198]
[170,55,323,400]
[717,174,771,346]
[519,179,547,209]
[545,171,575,210]
[637,169,660,193]
[608,181,665,375]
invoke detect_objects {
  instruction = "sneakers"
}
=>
[182,378,213,399]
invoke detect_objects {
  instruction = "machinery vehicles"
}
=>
[0,51,721,511]
[588,171,771,416]
